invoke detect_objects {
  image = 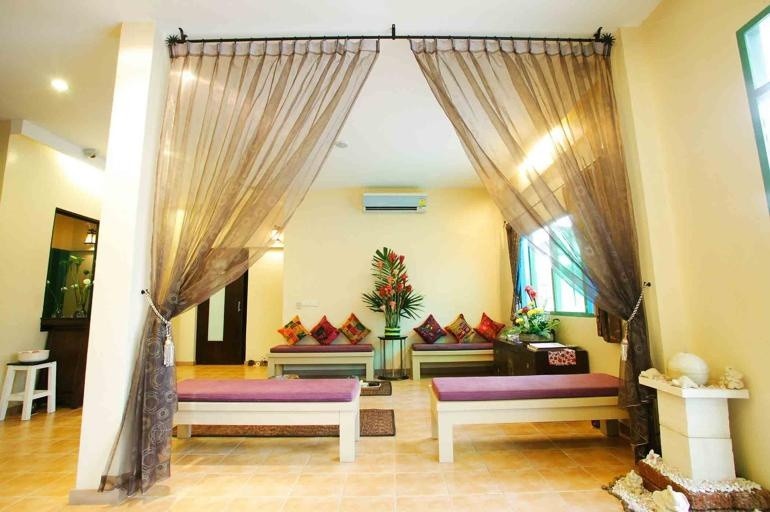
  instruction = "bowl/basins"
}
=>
[17,350,50,362]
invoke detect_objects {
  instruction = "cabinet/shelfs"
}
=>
[34,317,91,409]
[494,337,590,376]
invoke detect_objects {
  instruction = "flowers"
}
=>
[361,246,424,327]
[68,255,93,310]
[46,279,68,308]
[501,285,561,339]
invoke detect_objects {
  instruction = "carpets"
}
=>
[267,375,392,396]
[169,409,396,437]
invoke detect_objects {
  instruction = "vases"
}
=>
[518,333,555,342]
[72,310,87,319]
[51,308,64,318]
[385,327,400,338]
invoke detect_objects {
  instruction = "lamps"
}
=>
[83,221,97,244]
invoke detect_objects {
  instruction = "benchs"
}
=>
[428,372,633,462]
[173,378,362,462]
[267,344,375,381]
[410,341,494,381]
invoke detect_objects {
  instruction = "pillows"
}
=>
[338,313,371,345]
[278,315,310,346]
[444,313,472,344]
[473,312,506,342]
[309,315,340,346]
[413,313,447,345]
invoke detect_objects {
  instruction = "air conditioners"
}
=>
[362,192,428,213]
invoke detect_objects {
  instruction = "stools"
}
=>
[377,336,409,381]
[0,360,57,422]
